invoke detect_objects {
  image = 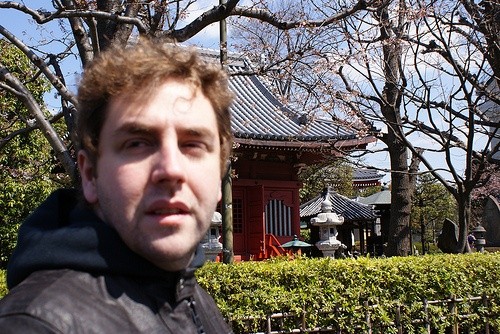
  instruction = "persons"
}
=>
[0,36,237,334]
